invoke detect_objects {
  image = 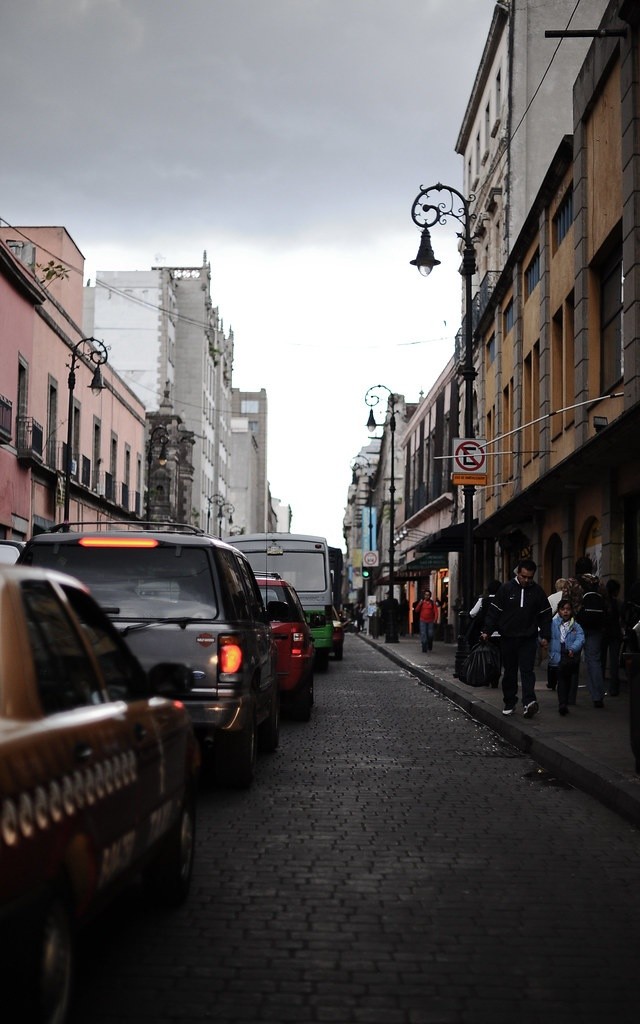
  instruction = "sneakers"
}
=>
[502,703,516,715]
[523,700,539,720]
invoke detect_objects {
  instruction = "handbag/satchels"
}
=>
[458,637,494,685]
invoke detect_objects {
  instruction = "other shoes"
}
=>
[593,700,605,709]
[558,705,569,716]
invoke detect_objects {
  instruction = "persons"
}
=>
[546,557,629,708]
[381,591,400,644]
[479,560,554,718]
[469,580,503,689]
[537,600,585,717]
[415,590,438,653]
[350,602,367,632]
[400,592,410,636]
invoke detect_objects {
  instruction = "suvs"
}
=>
[14,520,280,792]
[254,569,316,722]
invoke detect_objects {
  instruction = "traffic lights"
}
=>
[362,567,371,580]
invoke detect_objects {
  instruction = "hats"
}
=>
[384,589,390,593]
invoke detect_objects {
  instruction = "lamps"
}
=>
[393,527,431,546]
[593,416,608,433]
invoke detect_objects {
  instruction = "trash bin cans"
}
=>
[458,611,476,641]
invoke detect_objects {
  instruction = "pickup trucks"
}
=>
[0,561,210,1024]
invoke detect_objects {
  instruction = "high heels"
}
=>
[545,682,556,690]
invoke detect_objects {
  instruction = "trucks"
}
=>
[328,544,345,621]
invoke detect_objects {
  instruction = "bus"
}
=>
[222,530,335,673]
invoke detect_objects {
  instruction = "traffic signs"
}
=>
[451,438,488,486]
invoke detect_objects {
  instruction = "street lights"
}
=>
[350,454,378,597]
[407,177,489,678]
[204,492,245,539]
[364,382,401,643]
[144,422,172,529]
[63,336,113,532]
